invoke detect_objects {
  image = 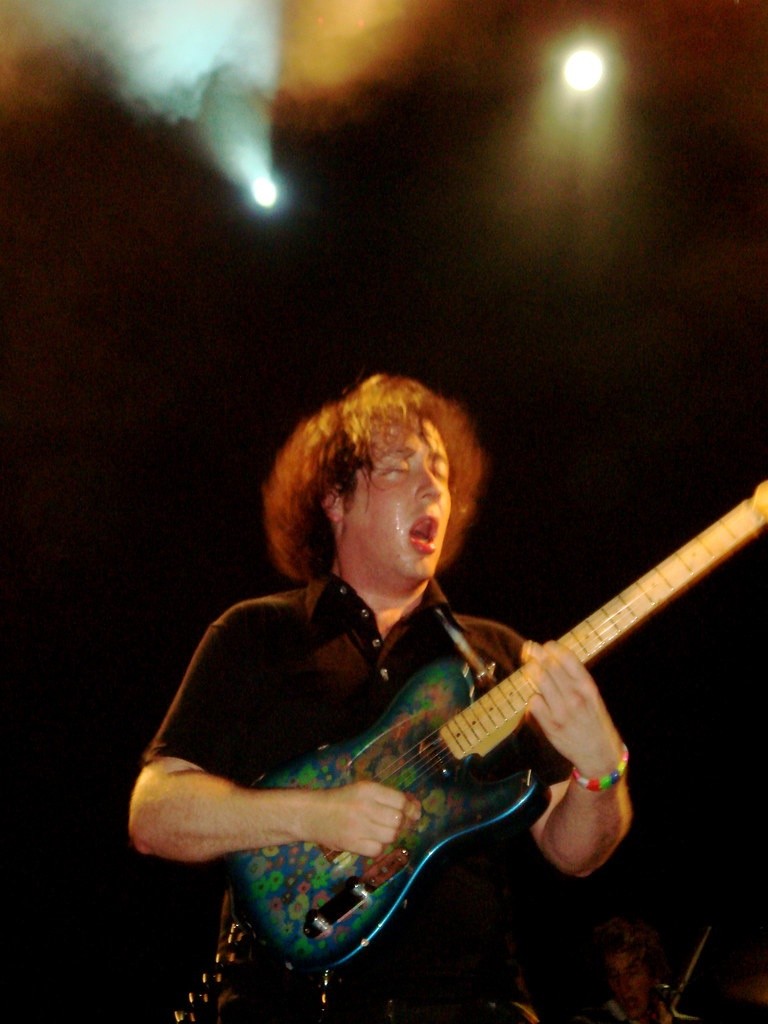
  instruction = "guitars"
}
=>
[226,477,767,970]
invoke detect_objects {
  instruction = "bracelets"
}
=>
[572,745,630,791]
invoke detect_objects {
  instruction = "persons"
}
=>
[126,376,633,1024]
[591,919,671,1024]
